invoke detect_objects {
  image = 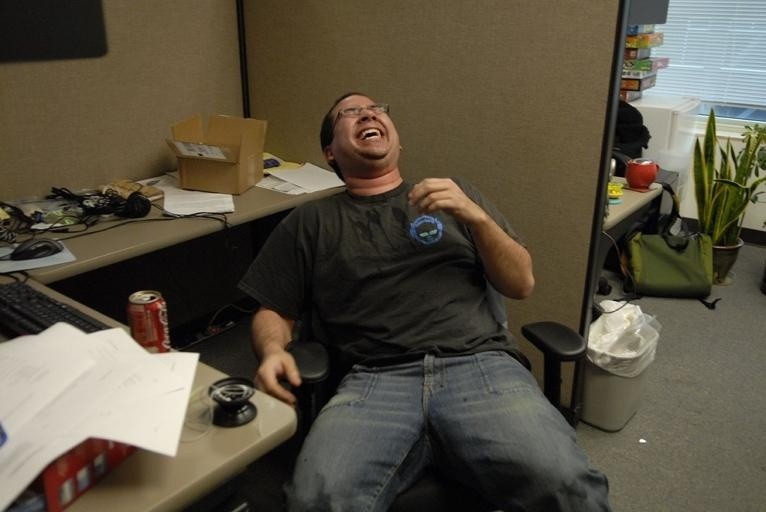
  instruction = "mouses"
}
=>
[10,238,64,260]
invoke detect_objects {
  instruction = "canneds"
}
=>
[128,290,171,352]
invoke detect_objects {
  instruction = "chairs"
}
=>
[268,214,589,511]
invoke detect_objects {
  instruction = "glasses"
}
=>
[332,103,390,131]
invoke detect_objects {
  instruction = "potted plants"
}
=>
[689,107,765,280]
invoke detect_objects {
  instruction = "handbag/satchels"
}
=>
[627,231,713,300]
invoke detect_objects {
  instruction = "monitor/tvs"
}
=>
[0,0,107,64]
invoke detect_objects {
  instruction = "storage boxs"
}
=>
[168,111,268,195]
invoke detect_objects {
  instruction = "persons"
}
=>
[236,94,612,512]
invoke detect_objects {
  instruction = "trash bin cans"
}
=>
[580,310,662,434]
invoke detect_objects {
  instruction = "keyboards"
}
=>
[0,281,113,336]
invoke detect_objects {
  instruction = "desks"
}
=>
[19,153,349,285]
[593,175,666,294]
[0,272,298,512]
